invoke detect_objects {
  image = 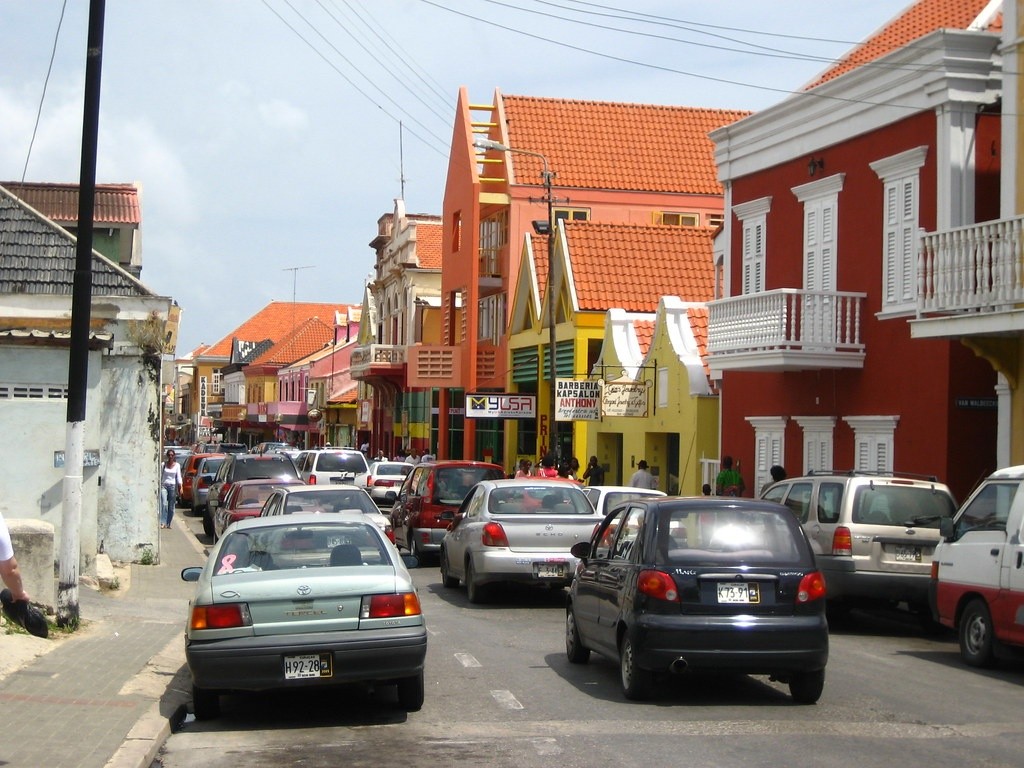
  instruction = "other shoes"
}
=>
[161,524,165,529]
[167,526,172,529]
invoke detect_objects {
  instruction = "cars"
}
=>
[440,479,609,601]
[260,486,395,567]
[567,485,687,560]
[191,456,225,514]
[180,511,427,719]
[212,478,306,548]
[370,462,415,506]
[566,493,829,703]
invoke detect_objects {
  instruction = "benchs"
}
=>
[498,502,576,513]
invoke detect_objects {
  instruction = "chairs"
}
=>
[284,505,303,514]
[330,544,363,566]
[542,494,558,508]
[495,490,510,503]
[248,550,274,568]
[334,504,363,514]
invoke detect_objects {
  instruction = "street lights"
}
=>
[470,137,558,469]
[308,317,335,391]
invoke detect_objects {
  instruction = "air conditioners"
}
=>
[175,413,187,423]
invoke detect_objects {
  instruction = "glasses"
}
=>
[168,454,175,457]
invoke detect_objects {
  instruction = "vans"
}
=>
[929,464,1024,667]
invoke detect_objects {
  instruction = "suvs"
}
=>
[163,445,226,508]
[295,445,373,494]
[387,460,505,566]
[194,442,248,455]
[203,451,299,533]
[250,442,300,454]
[761,468,958,626]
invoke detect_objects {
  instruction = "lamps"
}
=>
[532,219,550,234]
[808,156,824,177]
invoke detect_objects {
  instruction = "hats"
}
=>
[636,460,650,468]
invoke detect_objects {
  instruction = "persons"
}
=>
[630,459,656,490]
[161,450,183,529]
[703,484,711,495]
[0,512,30,604]
[374,448,435,465]
[583,456,604,486]
[513,456,584,483]
[360,440,369,453]
[715,456,745,498]
[761,465,786,496]
